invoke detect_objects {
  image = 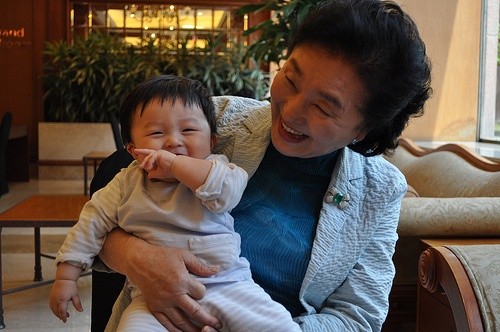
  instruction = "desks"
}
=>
[0,193,95,331]
[418,237,500,332]
[83,151,116,196]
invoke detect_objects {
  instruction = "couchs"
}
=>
[418,242,500,332]
[379,137,500,332]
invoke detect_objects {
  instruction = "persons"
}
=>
[90,0,434,330]
[48,75,302,332]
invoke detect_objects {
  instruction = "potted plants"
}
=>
[38,26,271,165]
